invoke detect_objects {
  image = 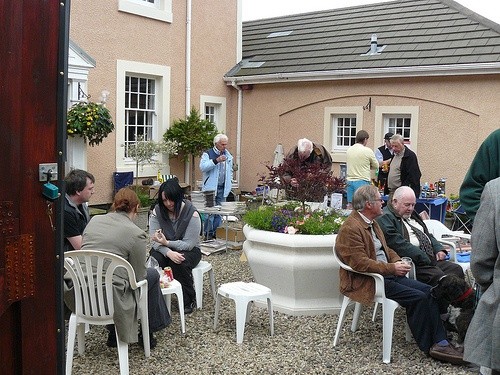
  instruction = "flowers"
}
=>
[66,101,114,147]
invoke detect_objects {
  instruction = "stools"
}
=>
[214,281,274,343]
[161,279,186,334]
[192,260,216,310]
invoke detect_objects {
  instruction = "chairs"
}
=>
[333,234,416,364]
[64,249,151,375]
[447,199,471,234]
[422,220,477,289]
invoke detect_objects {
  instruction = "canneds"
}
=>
[421,189,435,198]
[164,266,173,281]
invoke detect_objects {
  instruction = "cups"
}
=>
[421,178,448,198]
[401,257,412,265]
[383,161,389,173]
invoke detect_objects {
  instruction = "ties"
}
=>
[403,218,435,258]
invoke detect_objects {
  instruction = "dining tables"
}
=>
[342,194,448,225]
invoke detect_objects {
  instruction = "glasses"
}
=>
[369,199,383,203]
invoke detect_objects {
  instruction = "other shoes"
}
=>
[203,231,216,240]
[138,332,157,348]
[184,299,197,313]
[107,333,117,348]
[443,318,456,332]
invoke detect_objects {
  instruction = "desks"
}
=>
[146,183,191,231]
[199,210,247,253]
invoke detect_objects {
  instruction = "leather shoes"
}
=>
[430,341,470,365]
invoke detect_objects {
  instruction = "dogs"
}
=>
[429,274,479,349]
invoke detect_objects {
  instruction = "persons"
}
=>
[375,132,397,188]
[463,177,500,375]
[199,134,233,241]
[382,134,422,206]
[459,127,500,225]
[346,129,380,210]
[278,138,332,204]
[148,177,203,314]
[64,187,172,350]
[64,169,96,275]
[373,186,465,340]
[335,184,472,365]
